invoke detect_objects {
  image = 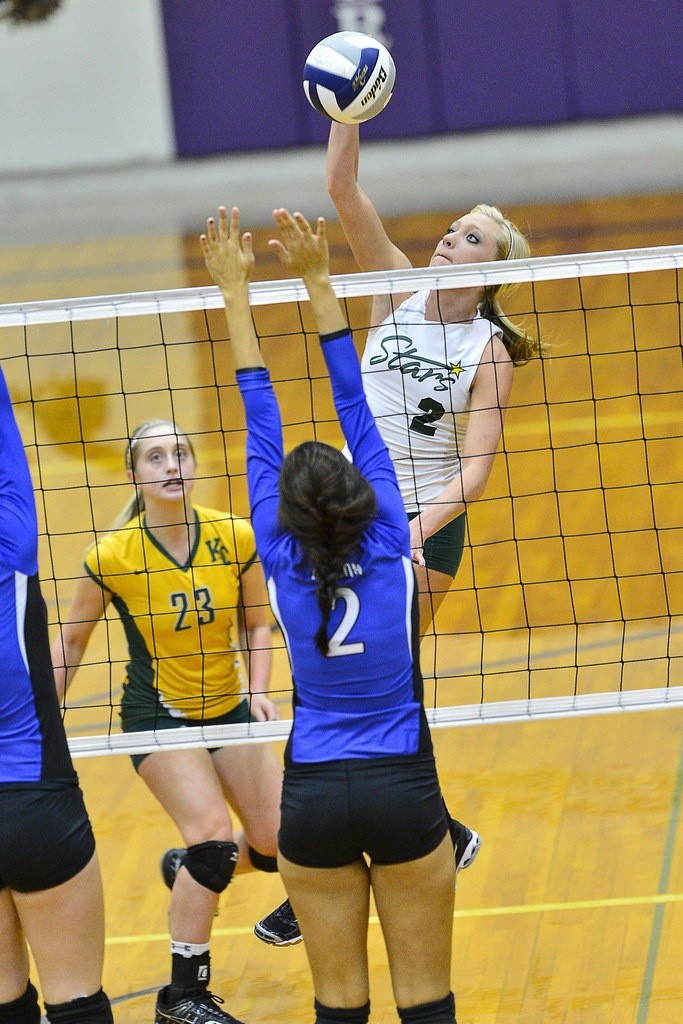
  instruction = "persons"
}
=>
[2,364,123,1024]
[49,419,289,1024]
[201,207,460,1024]
[251,122,554,947]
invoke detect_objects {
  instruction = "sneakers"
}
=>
[447,816,481,891]
[153,984,246,1024]
[253,895,303,948]
[159,848,189,893]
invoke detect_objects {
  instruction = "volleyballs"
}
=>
[301,31,396,125]
